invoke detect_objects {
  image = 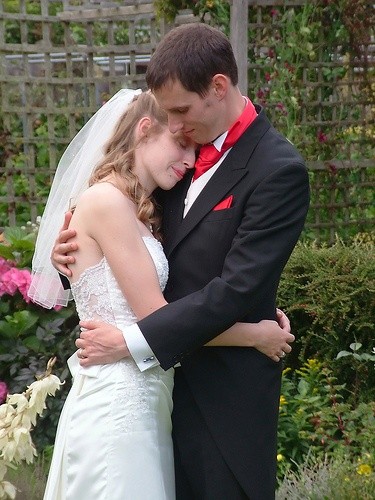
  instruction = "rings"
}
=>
[80,348,86,359]
[279,351,285,357]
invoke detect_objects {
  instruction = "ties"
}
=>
[193,96,256,182]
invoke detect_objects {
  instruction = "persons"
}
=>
[51,24,310,500]
[27,88,294,500]
